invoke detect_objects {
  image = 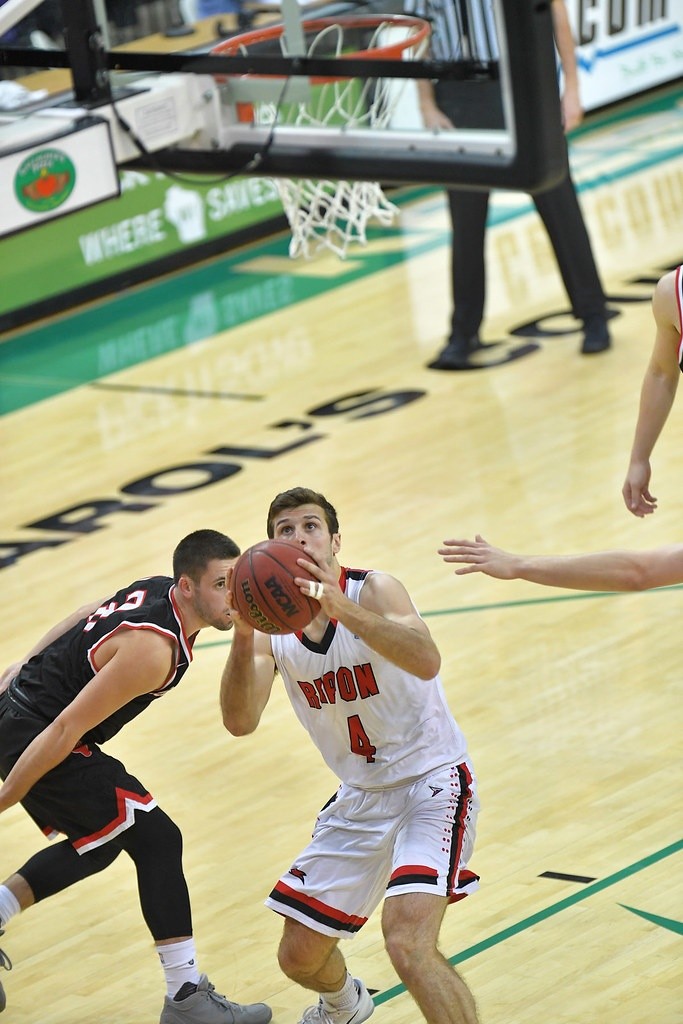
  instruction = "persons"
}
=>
[405,1,611,366]
[436,533,683,594]
[219,484,481,1024]
[0,528,273,1024]
[622,264,683,522]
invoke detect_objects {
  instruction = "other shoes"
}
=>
[581,313,612,353]
[437,330,480,364]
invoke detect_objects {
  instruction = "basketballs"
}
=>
[228,539,322,636]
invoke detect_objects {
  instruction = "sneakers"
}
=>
[160,974,272,1024]
[296,978,374,1024]
